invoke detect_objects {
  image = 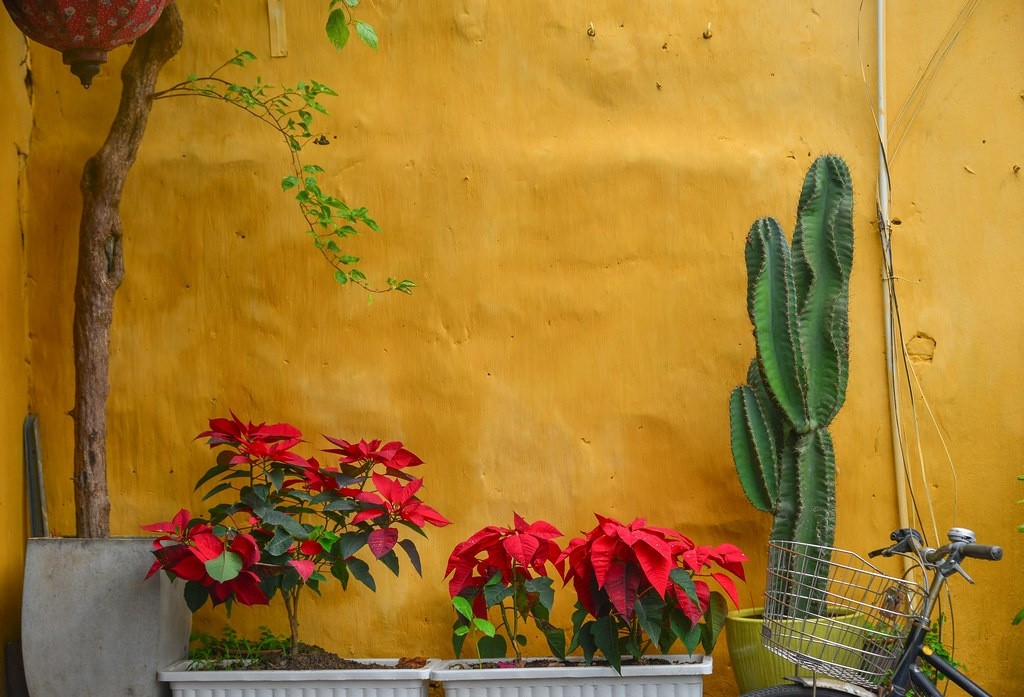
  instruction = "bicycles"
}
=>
[737,527,1005,697]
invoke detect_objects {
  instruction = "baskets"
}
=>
[761,540,930,689]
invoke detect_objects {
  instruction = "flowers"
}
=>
[441,511,750,670]
[140,408,448,654]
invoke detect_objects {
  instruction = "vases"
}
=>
[428,653,712,697]
[157,658,436,697]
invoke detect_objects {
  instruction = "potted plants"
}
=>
[722,154,864,691]
[21,0,416,697]
